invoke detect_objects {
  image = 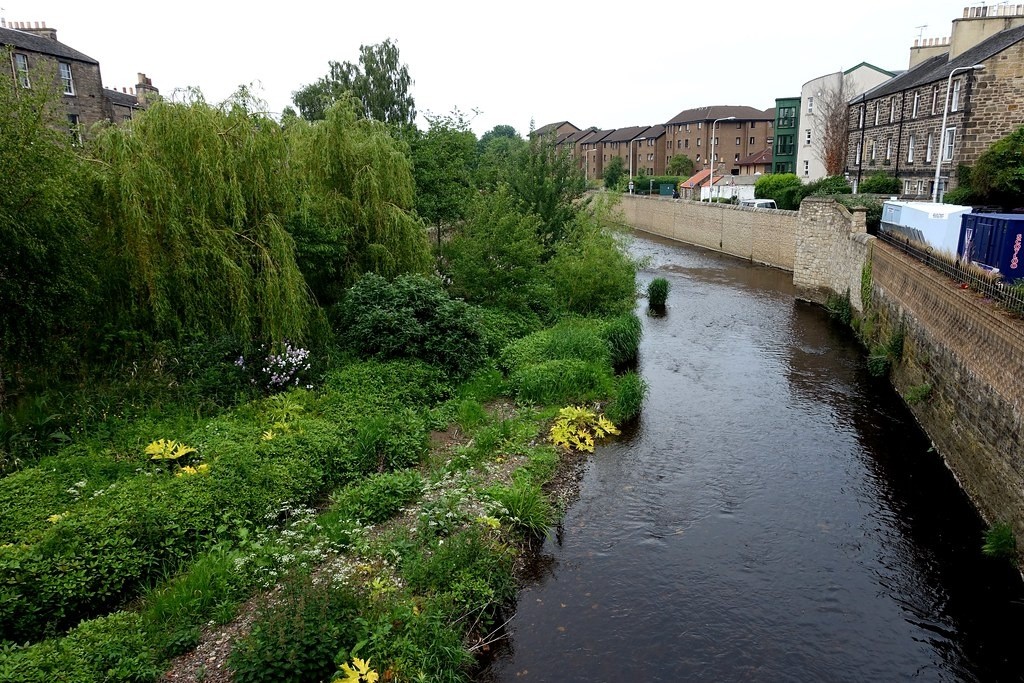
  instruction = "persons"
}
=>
[673,190,680,199]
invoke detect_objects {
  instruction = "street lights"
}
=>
[709,116,735,202]
[585,149,597,190]
[629,137,646,194]
[932,65,987,203]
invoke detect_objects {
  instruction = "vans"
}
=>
[736,199,778,210]
